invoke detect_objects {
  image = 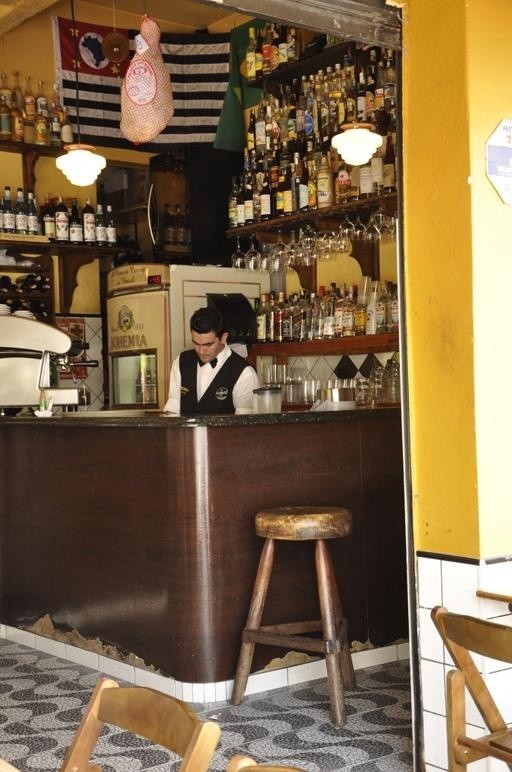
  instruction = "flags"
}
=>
[52,15,230,148]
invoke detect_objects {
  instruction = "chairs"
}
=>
[430,605,512,772]
[58,677,219,772]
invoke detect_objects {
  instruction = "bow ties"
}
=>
[198,357,217,368]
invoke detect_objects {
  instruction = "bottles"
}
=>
[83,198,96,245]
[3,186,16,233]
[106,205,117,246]
[174,204,184,244]
[41,192,56,243]
[69,199,83,244]
[79,385,90,412]
[228,50,397,227]
[0,188,4,233]
[240,21,295,81]
[12,72,24,110]
[23,75,37,119]
[35,111,49,146]
[95,204,107,246]
[252,386,282,414]
[50,116,61,147]
[254,276,398,342]
[183,205,193,244]
[161,203,174,244]
[61,106,75,147]
[11,92,24,142]
[15,188,27,235]
[27,192,38,235]
[33,197,43,235]
[35,79,49,117]
[50,82,67,123]
[0,73,13,109]
[0,96,11,140]
[54,196,70,244]
[1,274,53,292]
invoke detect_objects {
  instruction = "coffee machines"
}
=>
[0,314,100,415]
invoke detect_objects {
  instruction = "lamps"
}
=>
[332,39,383,166]
[55,0,106,187]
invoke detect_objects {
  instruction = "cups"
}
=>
[355,379,374,410]
[367,367,384,403]
[262,363,355,403]
[382,360,399,402]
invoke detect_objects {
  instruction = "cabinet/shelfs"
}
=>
[225,41,400,411]
[0,139,125,312]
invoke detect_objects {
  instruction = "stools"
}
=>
[231,507,356,727]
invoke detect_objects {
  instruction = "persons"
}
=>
[162,308,260,416]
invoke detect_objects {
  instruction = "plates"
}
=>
[0,303,11,314]
[12,310,34,317]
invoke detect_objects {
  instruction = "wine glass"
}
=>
[231,206,395,272]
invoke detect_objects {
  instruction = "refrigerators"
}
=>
[106,264,269,408]
